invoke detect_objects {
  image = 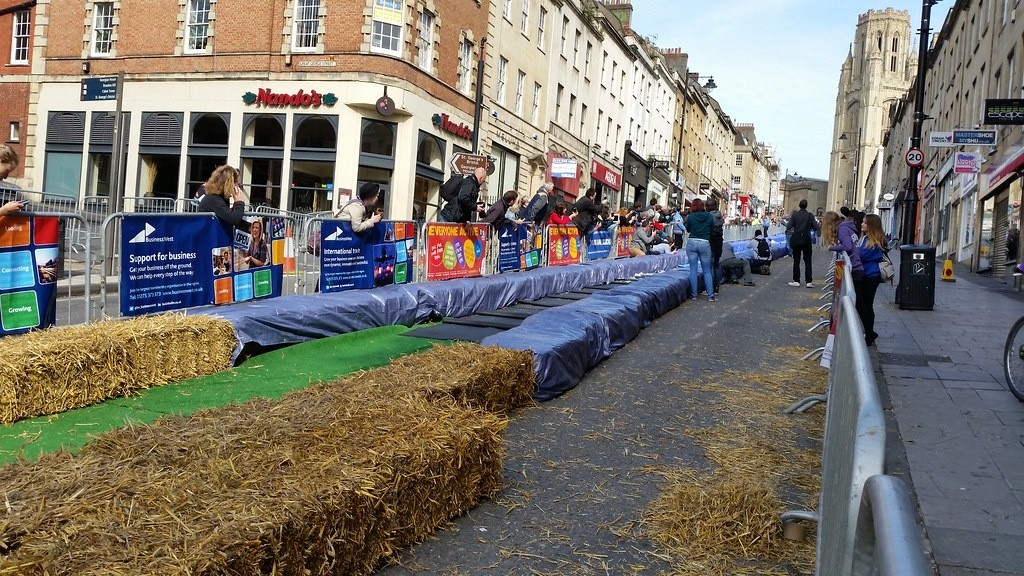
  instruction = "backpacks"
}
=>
[439,173,476,202]
[753,237,771,258]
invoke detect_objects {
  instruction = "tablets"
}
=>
[610,214,619,222]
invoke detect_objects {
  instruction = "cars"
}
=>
[0,181,24,207]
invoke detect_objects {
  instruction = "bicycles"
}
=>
[1004,315,1024,403]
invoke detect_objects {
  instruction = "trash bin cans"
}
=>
[898,244,936,311]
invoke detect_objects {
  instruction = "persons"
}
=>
[314,182,381,293]
[747,230,772,274]
[0,144,25,216]
[194,165,244,225]
[218,252,230,272]
[719,242,755,286]
[439,167,487,229]
[759,215,780,237]
[686,198,727,302]
[781,210,797,259]
[482,182,686,258]
[821,206,885,346]
[1008,224,1020,260]
[786,199,818,288]
[240,221,266,268]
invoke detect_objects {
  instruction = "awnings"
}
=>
[979,170,1023,201]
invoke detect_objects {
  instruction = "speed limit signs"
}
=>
[905,148,924,166]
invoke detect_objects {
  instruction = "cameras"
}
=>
[477,202,487,218]
[366,209,383,222]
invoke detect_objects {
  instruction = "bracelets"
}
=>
[249,256,252,260]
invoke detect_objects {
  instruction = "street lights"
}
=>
[782,171,798,215]
[838,131,861,210]
[841,154,857,210]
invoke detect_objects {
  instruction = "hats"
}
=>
[358,183,379,200]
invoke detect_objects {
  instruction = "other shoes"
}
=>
[732,279,739,284]
[708,295,719,301]
[788,281,800,287]
[864,331,878,346]
[744,282,755,286]
[690,296,697,300]
[806,282,815,288]
[713,289,719,295]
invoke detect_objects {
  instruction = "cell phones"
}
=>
[19,199,30,205]
[573,207,577,212]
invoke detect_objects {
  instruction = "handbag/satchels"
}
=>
[879,261,895,281]
[789,232,806,252]
[308,229,320,258]
